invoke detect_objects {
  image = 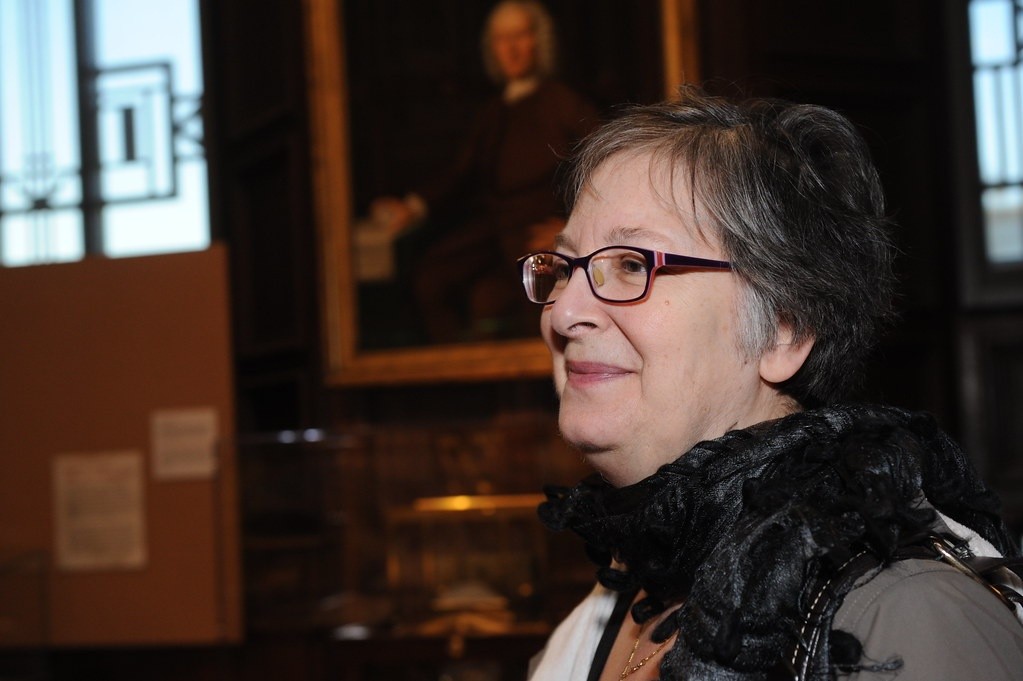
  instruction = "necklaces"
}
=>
[619,620,679,681]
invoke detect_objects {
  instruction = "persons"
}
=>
[516,86,1023,681]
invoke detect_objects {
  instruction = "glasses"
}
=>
[515,244,739,306]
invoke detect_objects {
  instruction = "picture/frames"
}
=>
[300,1,702,390]
